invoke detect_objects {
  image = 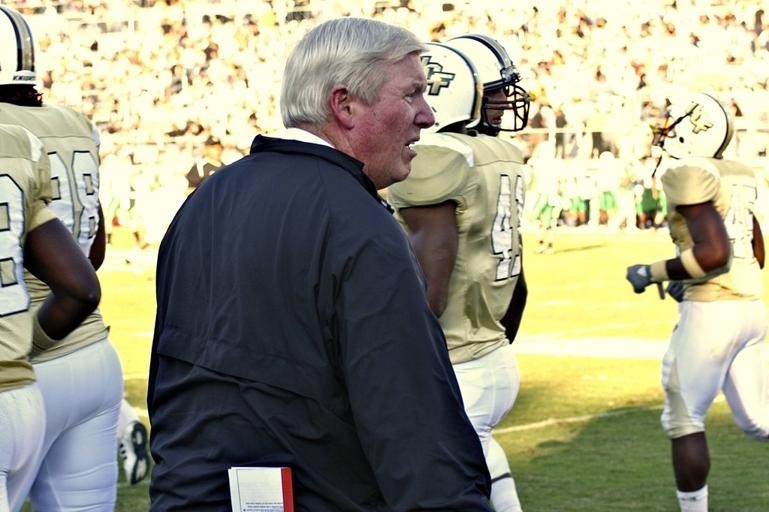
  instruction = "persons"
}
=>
[0,4,126,510]
[623,91,767,511]
[147,16,500,511]
[0,0,768,231]
[0,123,101,509]
[109,396,150,484]
[384,34,523,510]
[444,37,527,334]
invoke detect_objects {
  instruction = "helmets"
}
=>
[650,92,733,159]
[0,5,38,85]
[417,35,529,133]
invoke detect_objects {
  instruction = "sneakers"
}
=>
[119,421,149,485]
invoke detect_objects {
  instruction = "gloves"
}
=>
[627,265,654,293]
